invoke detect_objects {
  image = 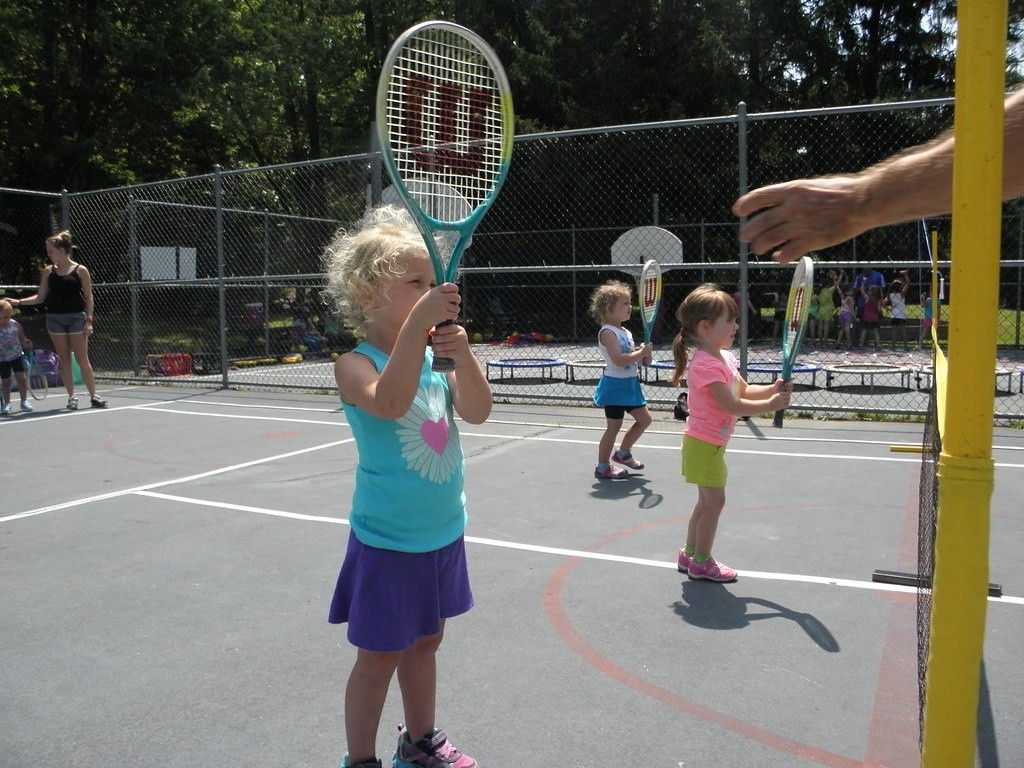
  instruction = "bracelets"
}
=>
[18,298,22,306]
[87,316,93,322]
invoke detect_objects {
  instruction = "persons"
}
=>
[724,282,789,350]
[731,84,1024,261]
[5,234,107,414]
[672,283,793,581]
[807,265,940,350]
[591,281,654,479]
[0,300,33,415]
[323,208,492,768]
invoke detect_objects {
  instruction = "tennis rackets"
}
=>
[638,256,662,362]
[27,340,48,401]
[375,20,517,371]
[771,255,817,429]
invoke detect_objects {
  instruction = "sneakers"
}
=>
[677,549,691,571]
[611,448,644,470]
[594,464,629,479]
[391,723,479,768]
[341,752,382,768]
[687,556,737,582]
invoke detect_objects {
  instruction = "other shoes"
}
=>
[2,404,12,412]
[20,400,33,411]
[91,395,110,409]
[67,396,78,409]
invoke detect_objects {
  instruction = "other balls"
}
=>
[472,333,483,343]
[331,352,339,362]
